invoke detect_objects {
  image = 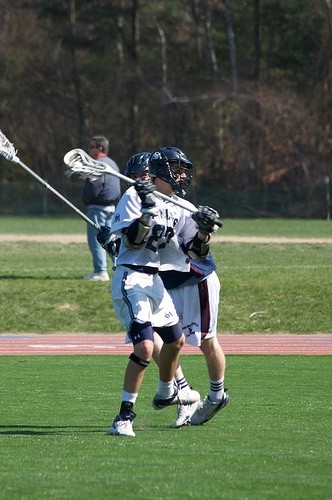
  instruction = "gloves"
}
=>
[135,177,160,216]
[97,226,117,255]
[192,205,219,235]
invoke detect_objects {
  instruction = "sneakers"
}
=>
[111,411,136,436]
[84,271,110,282]
[170,402,200,428]
[152,385,200,410]
[190,388,229,425]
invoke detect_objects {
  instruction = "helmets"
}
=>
[124,152,151,177]
[149,147,193,197]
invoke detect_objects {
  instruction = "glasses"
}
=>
[89,145,97,150]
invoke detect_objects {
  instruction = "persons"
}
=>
[107,146,220,438]
[83,136,122,283]
[98,152,230,428]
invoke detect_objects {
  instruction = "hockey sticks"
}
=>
[63,148,223,228]
[0,129,103,232]
